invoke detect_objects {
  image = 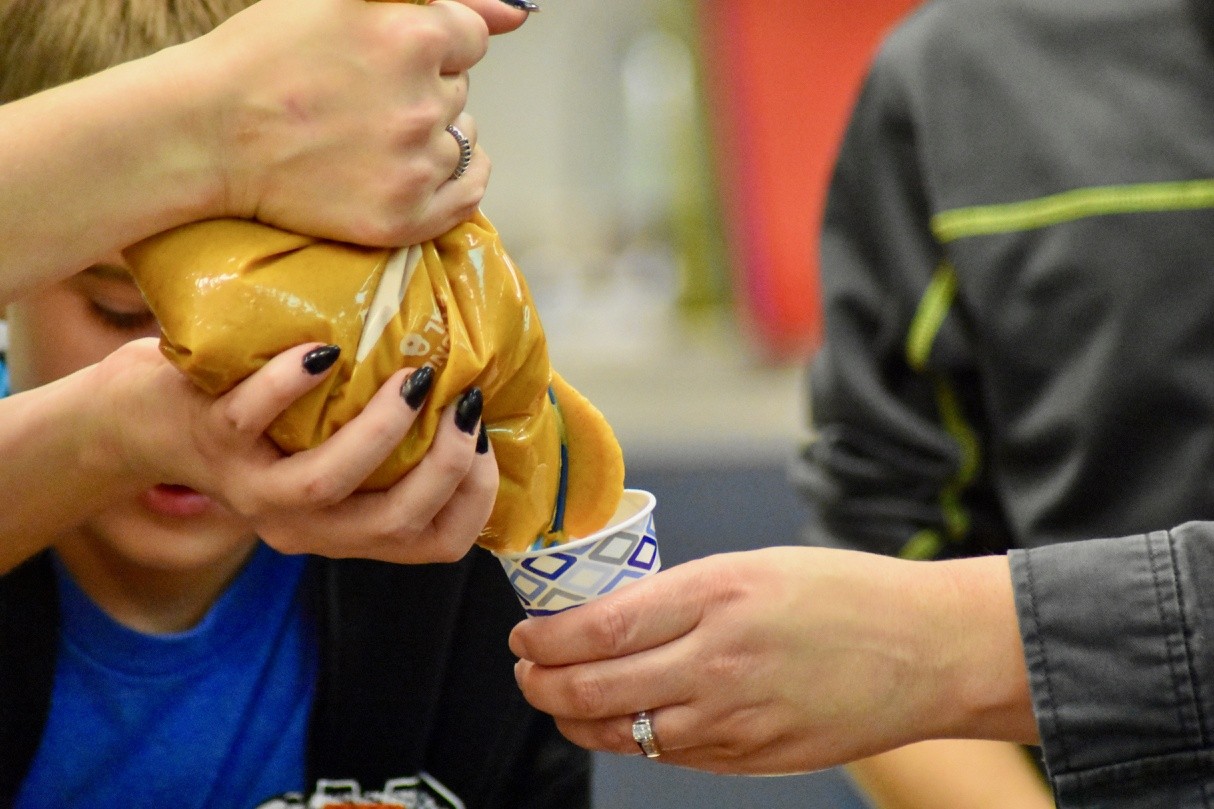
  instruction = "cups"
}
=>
[491,489,662,622]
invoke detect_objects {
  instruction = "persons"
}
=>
[1,0,1214,808]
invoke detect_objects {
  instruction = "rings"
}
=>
[446,124,472,181]
[632,711,662,759]
